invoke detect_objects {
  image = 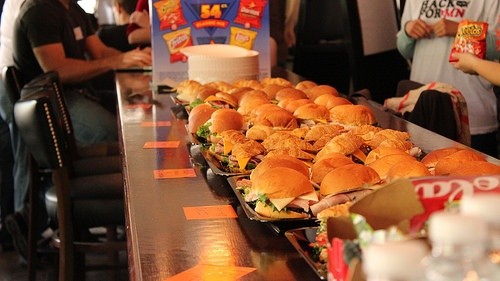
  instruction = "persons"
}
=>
[451,51,500,137]
[283,0,353,98]
[96,0,138,52]
[395,0,500,160]
[9,0,151,147]
[0,0,65,267]
[126,0,151,46]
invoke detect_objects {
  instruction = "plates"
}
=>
[180,44,260,85]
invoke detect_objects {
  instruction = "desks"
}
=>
[114,64,500,281]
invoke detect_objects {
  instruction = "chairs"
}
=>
[292,44,351,96]
[394,80,471,148]
[0,66,128,281]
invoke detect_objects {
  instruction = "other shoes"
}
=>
[5,212,45,269]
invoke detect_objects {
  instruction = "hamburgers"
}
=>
[176,78,317,103]
[308,201,360,273]
[188,85,376,141]
[235,147,500,219]
[207,125,412,172]
